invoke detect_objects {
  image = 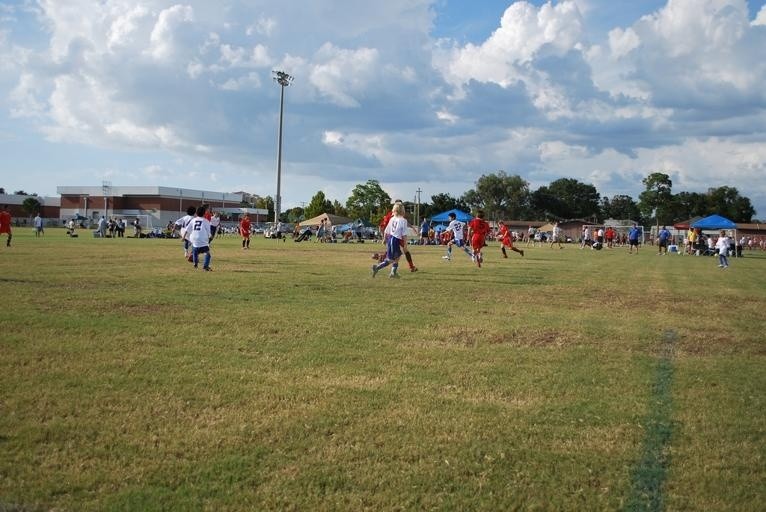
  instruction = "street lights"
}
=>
[272,67,295,223]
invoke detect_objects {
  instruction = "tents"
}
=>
[430,209,474,232]
[690,214,738,258]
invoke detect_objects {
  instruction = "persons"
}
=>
[372,200,418,278]
[355,220,362,242]
[656,226,763,271]
[69,219,74,232]
[167,204,255,272]
[421,211,563,268]
[581,223,642,254]
[0,204,14,246]
[34,212,42,237]
[293,217,336,243]
[264,222,282,238]
[98,216,142,237]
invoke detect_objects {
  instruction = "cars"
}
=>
[251,224,263,234]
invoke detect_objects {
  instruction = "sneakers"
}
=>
[473,253,483,268]
[193,263,212,272]
[372,264,378,278]
[410,267,418,272]
[501,249,525,258]
[389,273,400,278]
[718,263,729,269]
